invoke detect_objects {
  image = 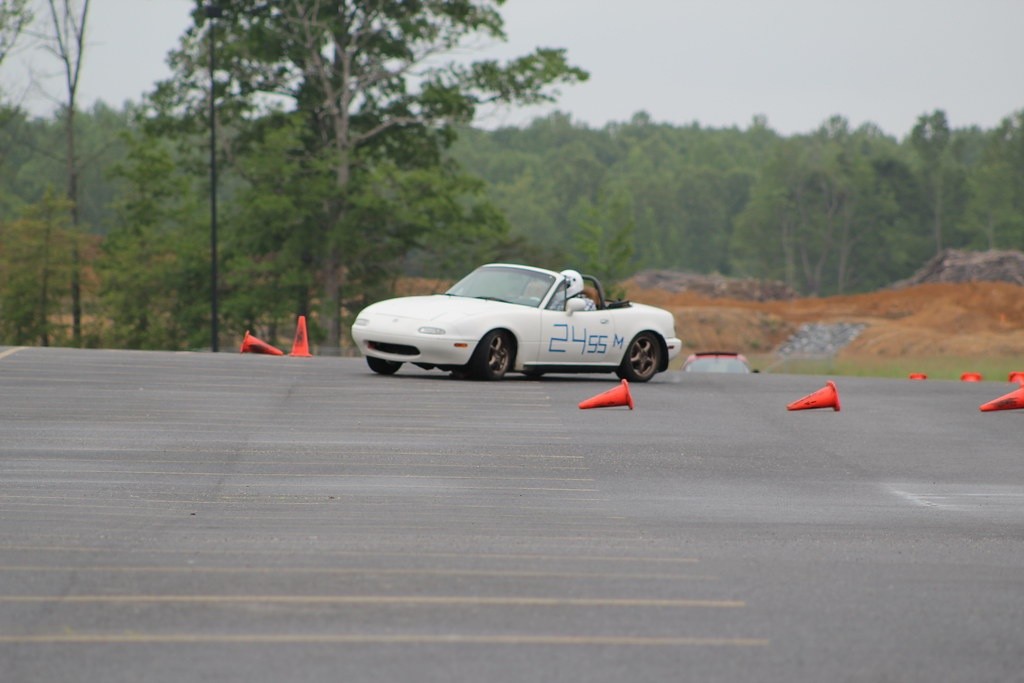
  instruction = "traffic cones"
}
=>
[287,315,313,356]
[960,371,983,382]
[1009,372,1024,385]
[785,380,842,412]
[980,381,1024,411]
[239,330,284,356]
[577,379,634,411]
[909,372,928,381]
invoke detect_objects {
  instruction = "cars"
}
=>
[679,349,760,374]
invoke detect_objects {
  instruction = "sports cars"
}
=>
[349,261,682,383]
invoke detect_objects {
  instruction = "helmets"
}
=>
[555,269,584,302]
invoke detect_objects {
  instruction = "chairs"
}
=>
[582,287,602,309]
[523,280,547,301]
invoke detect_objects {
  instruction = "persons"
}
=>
[547,269,596,311]
